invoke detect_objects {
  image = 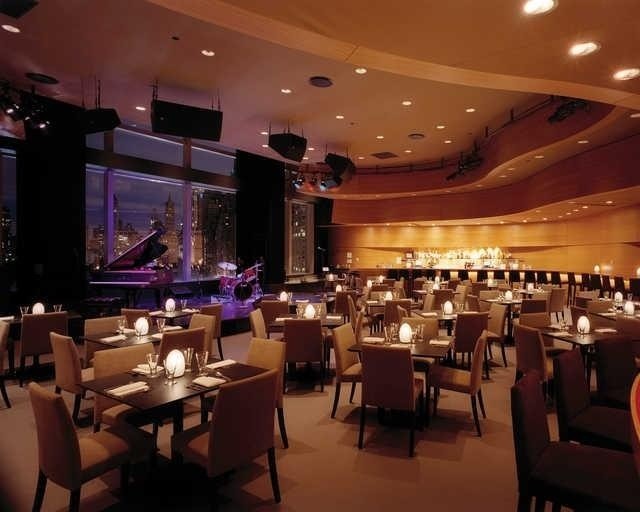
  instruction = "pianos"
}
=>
[89,231,174,309]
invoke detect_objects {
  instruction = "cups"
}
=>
[20,306,29,320]
[145,353,159,378]
[384,322,399,346]
[156,318,166,333]
[180,299,187,309]
[54,305,62,312]
[180,347,193,372]
[118,319,126,330]
[163,359,177,386]
[195,351,209,376]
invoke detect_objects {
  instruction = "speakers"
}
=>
[325,153,356,181]
[325,177,342,188]
[151,100,222,142]
[85,108,121,134]
[269,134,306,161]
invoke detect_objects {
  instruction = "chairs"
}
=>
[173,368,281,503]
[27,382,130,511]
[361,344,426,456]
[261,279,639,436]
[1,277,291,449]
[511,371,638,510]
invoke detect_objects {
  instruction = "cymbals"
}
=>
[218,263,236,271]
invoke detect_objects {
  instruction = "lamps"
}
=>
[268,120,307,162]
[79,80,121,135]
[150,80,223,143]
[4,84,50,129]
[292,170,331,190]
[325,144,356,179]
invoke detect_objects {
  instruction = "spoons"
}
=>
[215,371,231,381]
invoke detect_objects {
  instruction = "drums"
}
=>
[220,276,235,287]
[230,279,251,301]
[243,268,256,281]
